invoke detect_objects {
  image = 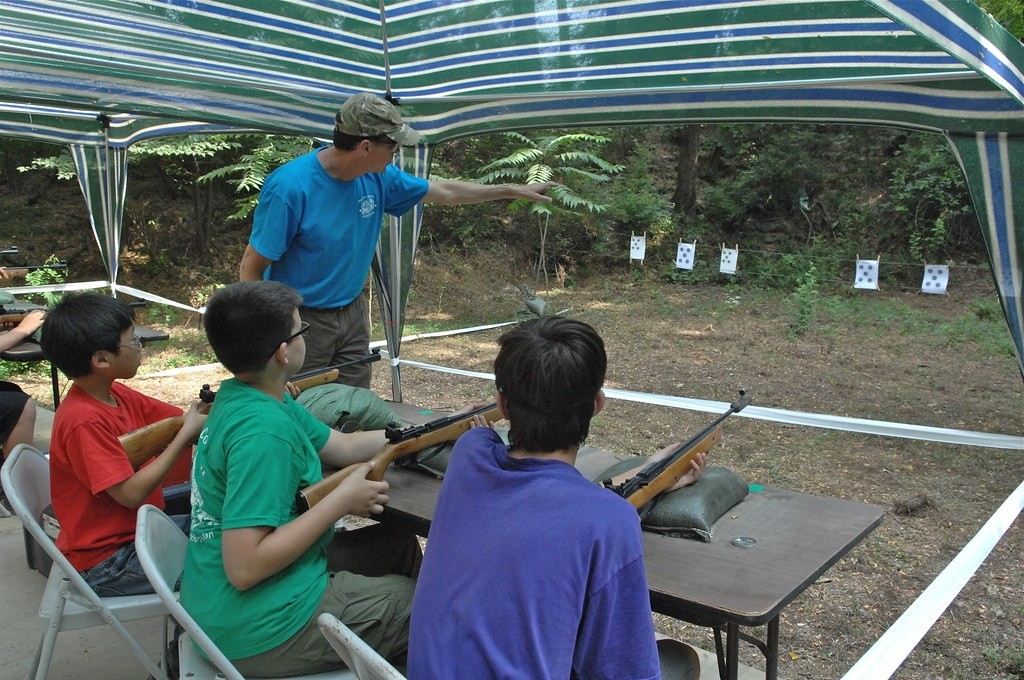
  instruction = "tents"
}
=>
[0,0,1024,401]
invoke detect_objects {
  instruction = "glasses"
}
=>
[267,321,312,360]
[99,334,141,351]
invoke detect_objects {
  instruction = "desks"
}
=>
[0,299,169,413]
[323,400,884,680]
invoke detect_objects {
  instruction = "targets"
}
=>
[916,260,949,296]
[851,253,881,292]
[672,238,697,270]
[629,230,646,266]
[718,242,739,275]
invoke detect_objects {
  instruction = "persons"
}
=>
[240,92,559,390]
[176,281,496,680]
[407,315,707,680]
[0,264,45,510]
[40,294,301,680]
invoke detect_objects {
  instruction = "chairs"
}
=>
[134,505,358,680]
[0,443,181,680]
[317,613,407,680]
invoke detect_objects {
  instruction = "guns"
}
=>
[1,259,67,279]
[294,401,505,513]
[117,345,388,473]
[0,301,148,324]
[602,388,754,512]
[2,243,21,253]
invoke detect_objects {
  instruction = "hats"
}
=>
[333,92,420,147]
